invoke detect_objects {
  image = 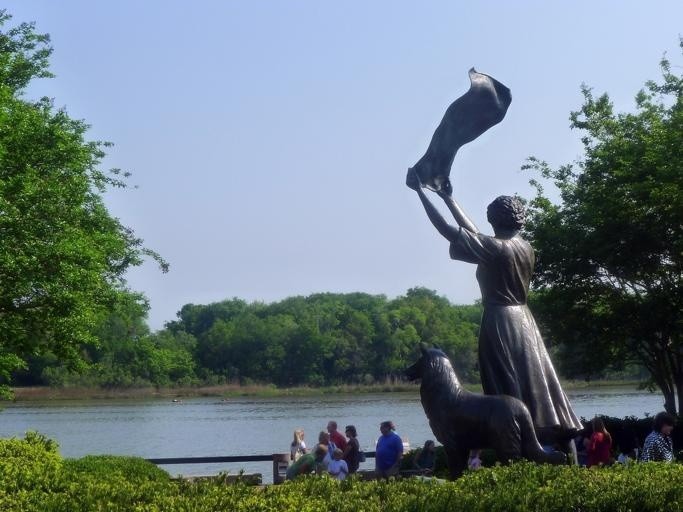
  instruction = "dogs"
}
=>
[406,348,566,482]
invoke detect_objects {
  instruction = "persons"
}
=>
[468,449,482,469]
[409,440,436,476]
[285,420,360,482]
[641,411,675,462]
[406,167,584,466]
[375,420,403,480]
[583,418,612,469]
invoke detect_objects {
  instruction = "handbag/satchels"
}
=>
[359,452,365,462]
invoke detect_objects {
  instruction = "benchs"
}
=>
[183,472,262,486]
[357,469,418,482]
